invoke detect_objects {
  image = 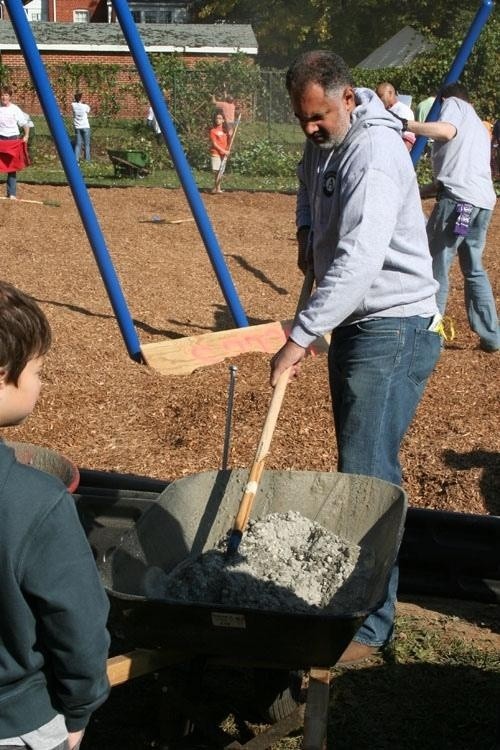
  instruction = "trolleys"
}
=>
[88,464,409,749]
[105,146,154,180]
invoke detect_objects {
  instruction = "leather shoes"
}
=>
[337,642,382,664]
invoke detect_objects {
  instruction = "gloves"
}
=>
[386,109,407,132]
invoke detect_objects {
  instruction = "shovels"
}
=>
[197,260,315,570]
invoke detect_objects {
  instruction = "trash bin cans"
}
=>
[107,149,149,180]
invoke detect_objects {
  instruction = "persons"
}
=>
[0,280,112,750]
[270,50,440,666]
[0,87,35,200]
[209,93,235,137]
[71,93,91,163]
[385,83,500,353]
[414,87,436,157]
[209,112,230,194]
[147,107,164,146]
[376,81,416,152]
